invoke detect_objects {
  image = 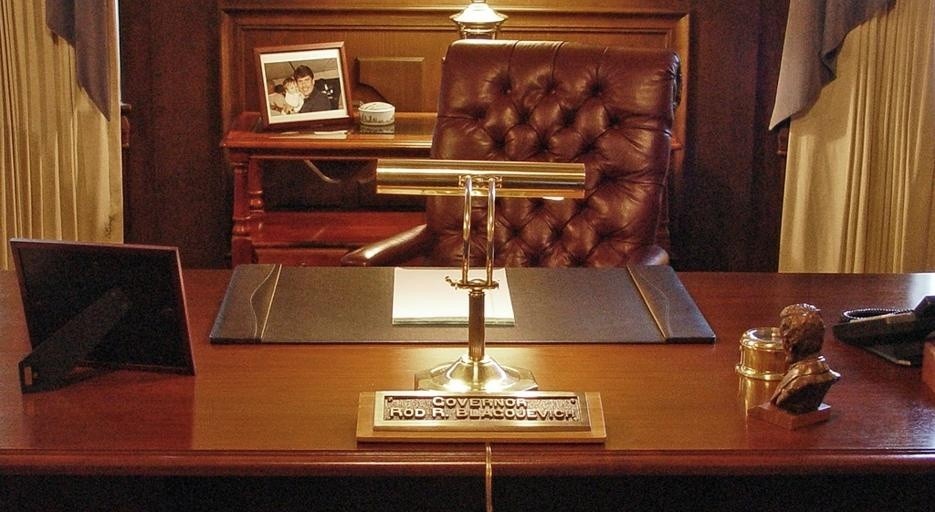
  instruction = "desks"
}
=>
[0,263,934,510]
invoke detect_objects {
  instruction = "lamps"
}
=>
[445,0,513,43]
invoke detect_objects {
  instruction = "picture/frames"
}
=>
[250,41,355,128]
[10,237,198,379]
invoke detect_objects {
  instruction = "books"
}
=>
[392,266,516,327]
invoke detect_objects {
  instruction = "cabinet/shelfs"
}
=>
[220,112,439,263]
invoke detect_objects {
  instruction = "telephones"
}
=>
[832,296,935,344]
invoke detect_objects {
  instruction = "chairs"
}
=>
[338,36,681,273]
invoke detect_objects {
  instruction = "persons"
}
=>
[267,65,330,116]
